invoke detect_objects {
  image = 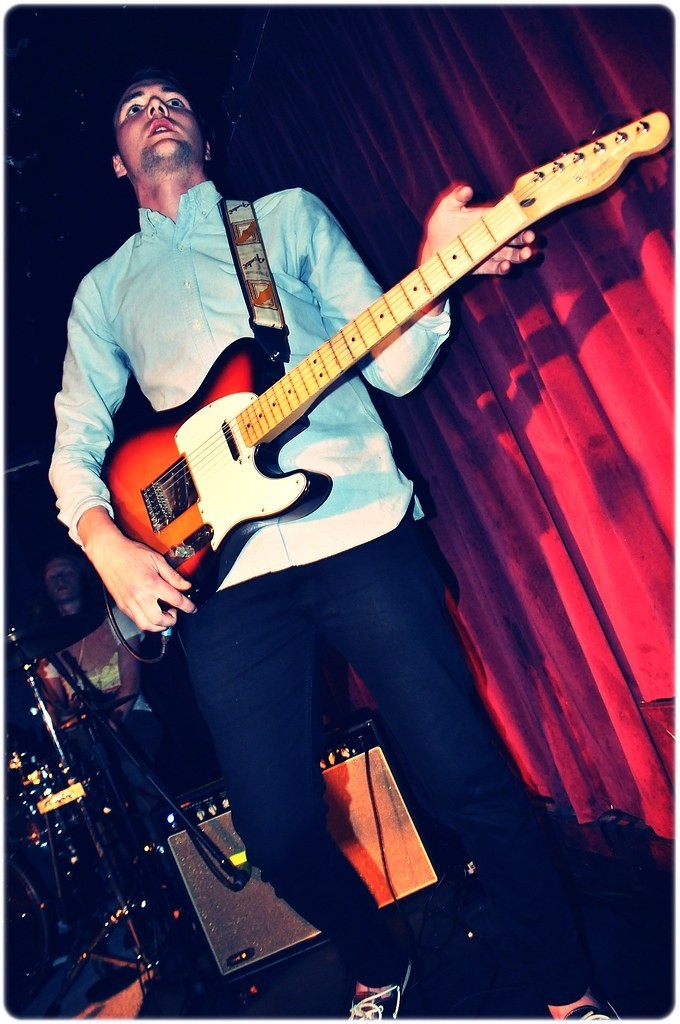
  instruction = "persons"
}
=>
[50,74,624,1018]
[33,547,174,892]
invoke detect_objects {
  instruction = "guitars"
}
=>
[104,111,670,660]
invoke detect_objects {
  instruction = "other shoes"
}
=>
[561,1001,619,1020]
[348,959,412,1020]
